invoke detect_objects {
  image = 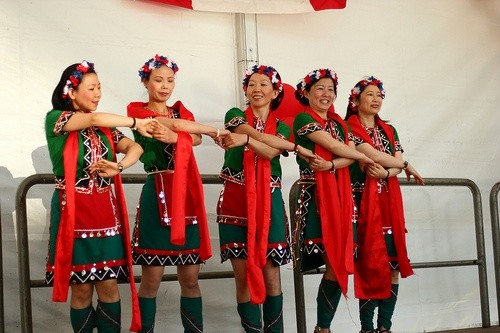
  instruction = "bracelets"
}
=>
[292,143,298,155]
[329,160,335,174]
[243,133,250,152]
[117,162,123,172]
[211,128,220,139]
[129,117,136,131]
[385,169,389,179]
[402,160,408,170]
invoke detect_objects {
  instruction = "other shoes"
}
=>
[358,327,378,333]
[375,326,392,333]
[312,325,331,333]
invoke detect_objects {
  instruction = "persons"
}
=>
[294,68,377,333]
[126,53,232,333]
[344,75,424,333]
[44,60,159,333]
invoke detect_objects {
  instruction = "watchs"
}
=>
[215,65,317,333]
